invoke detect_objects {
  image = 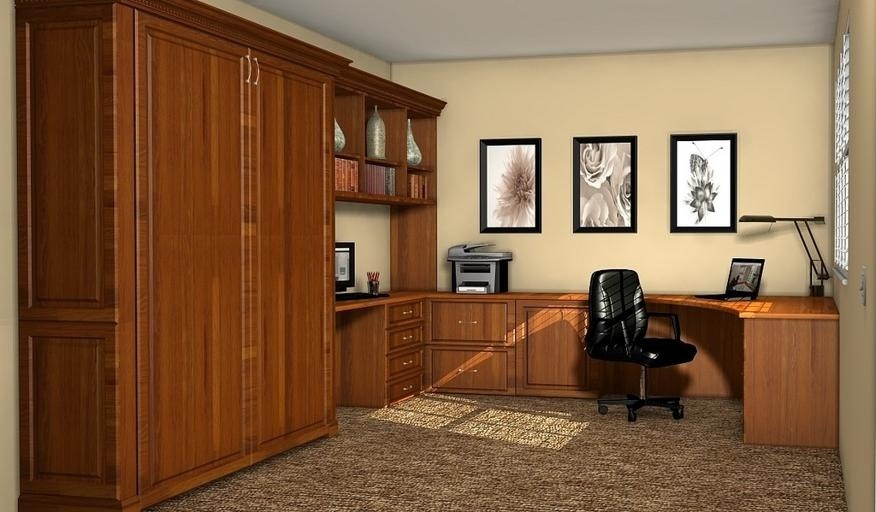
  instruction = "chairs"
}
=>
[586,268,698,422]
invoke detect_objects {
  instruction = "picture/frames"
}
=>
[669,132,740,233]
[572,136,638,234]
[478,139,542,233]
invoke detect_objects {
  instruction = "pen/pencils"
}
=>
[367,272,379,295]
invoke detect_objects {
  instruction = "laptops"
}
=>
[694,257,765,301]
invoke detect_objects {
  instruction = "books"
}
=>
[333,157,428,201]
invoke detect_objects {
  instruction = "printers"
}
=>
[448,244,512,293]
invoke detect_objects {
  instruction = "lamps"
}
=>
[739,213,831,297]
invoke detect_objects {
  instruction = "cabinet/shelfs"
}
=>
[337,299,423,409]
[515,298,604,399]
[422,298,515,398]
[15,1,353,510]
[332,67,448,205]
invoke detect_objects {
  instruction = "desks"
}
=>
[644,293,838,449]
